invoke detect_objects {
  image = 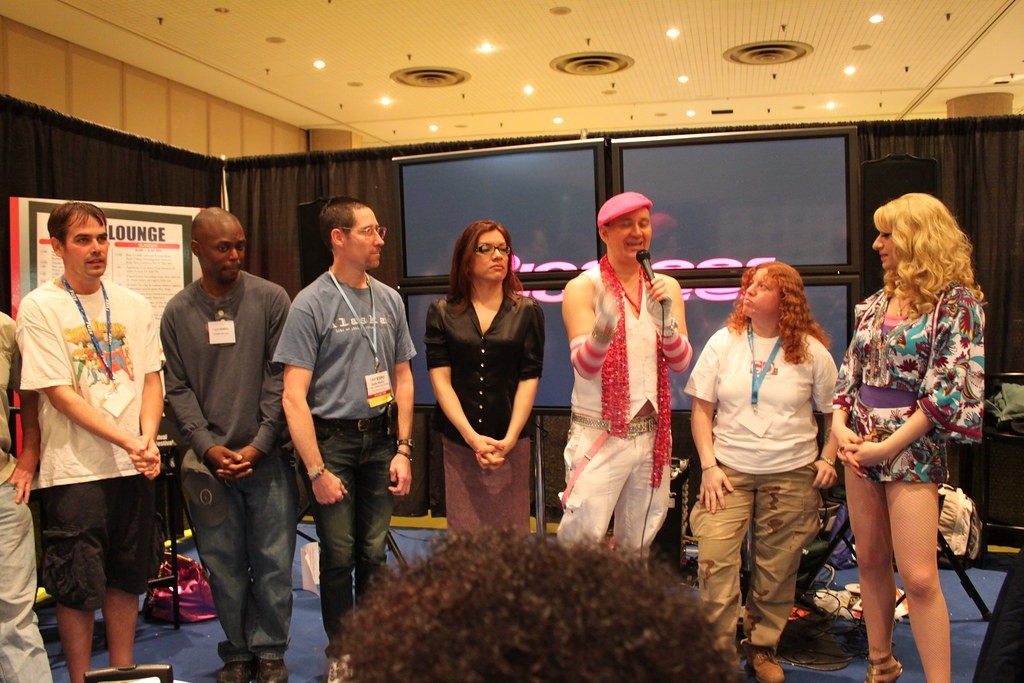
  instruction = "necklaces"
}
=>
[897,295,910,315]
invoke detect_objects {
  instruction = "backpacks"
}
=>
[936,483,981,567]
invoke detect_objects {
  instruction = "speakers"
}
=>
[298,199,332,289]
[861,152,937,299]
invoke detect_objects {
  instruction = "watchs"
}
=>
[821,455,836,466]
[660,326,677,338]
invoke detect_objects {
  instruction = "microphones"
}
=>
[636,249,664,304]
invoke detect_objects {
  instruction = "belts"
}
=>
[312,405,389,431]
[571,413,657,434]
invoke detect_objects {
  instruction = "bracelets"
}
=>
[592,326,613,343]
[307,464,328,481]
[397,440,418,447]
[700,464,718,470]
[397,450,414,462]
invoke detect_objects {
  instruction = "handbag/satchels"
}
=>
[144,552,218,623]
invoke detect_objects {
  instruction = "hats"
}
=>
[598,192,652,227]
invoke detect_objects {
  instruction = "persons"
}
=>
[650,213,708,410]
[513,228,549,262]
[0,310,53,683]
[683,262,840,683]
[14,203,167,683]
[556,191,693,569]
[272,193,419,682]
[324,531,738,683]
[424,221,544,535]
[832,192,987,683]
[158,207,291,683]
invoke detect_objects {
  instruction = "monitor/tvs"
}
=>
[392,125,862,416]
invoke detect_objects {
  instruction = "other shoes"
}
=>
[864,652,903,683]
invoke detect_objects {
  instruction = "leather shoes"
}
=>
[256,655,288,683]
[217,660,254,683]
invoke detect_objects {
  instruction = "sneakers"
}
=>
[743,642,784,683]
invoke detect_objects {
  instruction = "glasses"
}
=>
[475,244,511,256]
[335,227,387,239]
